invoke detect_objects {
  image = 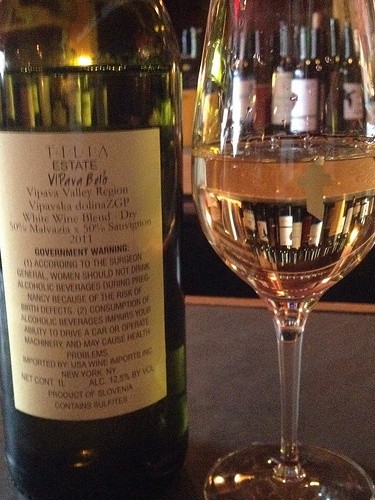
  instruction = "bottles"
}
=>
[0,0,190,500]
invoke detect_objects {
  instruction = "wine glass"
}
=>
[202,0,375,500]
